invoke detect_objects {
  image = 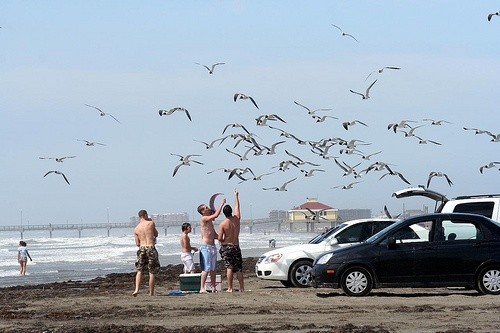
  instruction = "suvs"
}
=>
[392,188,500,240]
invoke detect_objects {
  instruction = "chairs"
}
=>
[448,232,457,240]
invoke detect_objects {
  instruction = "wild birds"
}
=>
[75,138,106,147]
[83,103,120,123]
[349,78,378,100]
[487,10,500,22]
[366,64,401,79]
[38,157,76,163]
[157,92,500,224]
[43,170,71,186]
[192,61,226,75]
[330,23,359,44]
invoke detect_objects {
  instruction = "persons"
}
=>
[132,210,160,296]
[196,199,226,294]
[18,241,32,275]
[269,239,276,248]
[180,223,198,274]
[219,188,244,293]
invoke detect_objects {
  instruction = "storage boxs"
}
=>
[179,273,201,291]
[204,274,222,291]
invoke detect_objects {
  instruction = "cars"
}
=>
[311,213,500,297]
[255,218,430,288]
